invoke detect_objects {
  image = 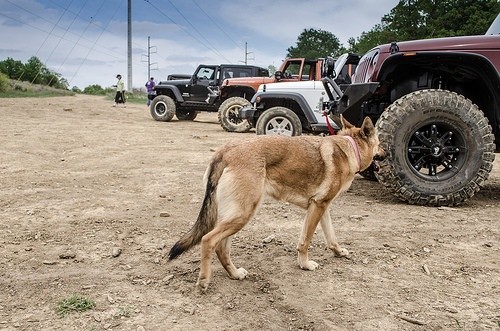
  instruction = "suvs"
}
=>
[216,55,357,131]
[238,53,368,137]
[320,11,500,206]
[147,64,270,122]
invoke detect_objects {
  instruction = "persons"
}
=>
[145,77,154,106]
[111,75,128,108]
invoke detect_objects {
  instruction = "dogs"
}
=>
[167,113,391,294]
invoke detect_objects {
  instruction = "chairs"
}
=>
[225,72,231,78]
